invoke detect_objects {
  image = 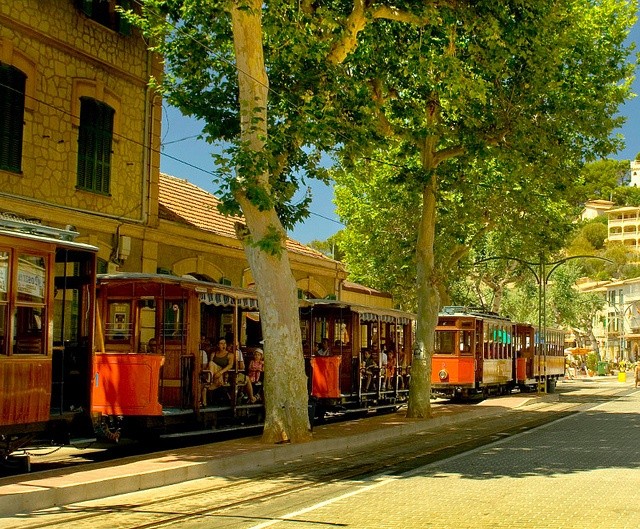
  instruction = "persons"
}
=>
[381,350,396,389]
[242,348,264,395]
[235,346,260,403]
[315,338,333,358]
[200,335,233,408]
[335,340,345,347]
[149,338,158,353]
[357,349,377,392]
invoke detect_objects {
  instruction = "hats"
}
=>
[253,348,263,359]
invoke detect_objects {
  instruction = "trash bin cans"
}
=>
[547,379,555,393]
